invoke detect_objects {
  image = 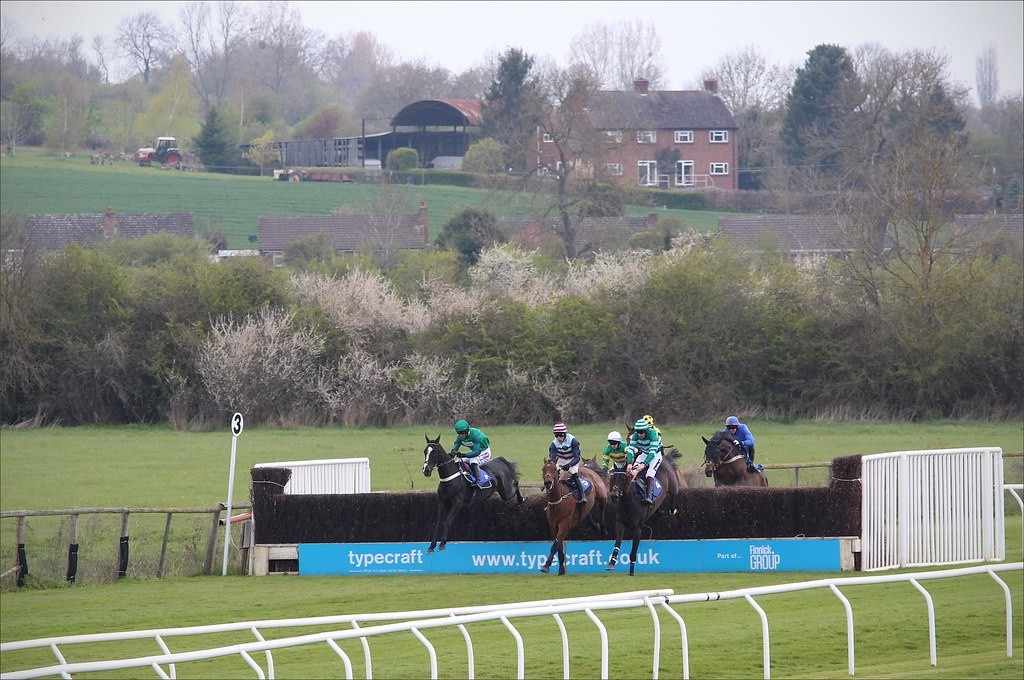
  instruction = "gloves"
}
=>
[733,440,739,445]
[561,464,570,471]
[448,453,456,458]
[456,452,466,458]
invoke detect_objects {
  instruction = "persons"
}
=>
[723,416,755,474]
[625,419,662,505]
[450,419,492,485]
[547,421,589,505]
[601,431,631,473]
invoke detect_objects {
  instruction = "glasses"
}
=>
[609,441,618,445]
[636,430,645,434]
[726,425,737,429]
[555,434,564,438]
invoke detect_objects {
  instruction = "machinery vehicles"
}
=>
[137,137,182,169]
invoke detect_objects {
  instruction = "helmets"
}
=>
[725,416,739,425]
[553,421,568,433]
[454,420,470,433]
[607,431,622,441]
[634,419,649,431]
[643,415,654,428]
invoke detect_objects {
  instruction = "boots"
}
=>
[473,466,483,489]
[645,478,655,505]
[573,474,587,503]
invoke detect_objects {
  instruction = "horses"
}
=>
[422,433,523,553]
[581,423,688,489]
[702,431,768,487]
[541,456,608,576]
[606,448,682,578]
